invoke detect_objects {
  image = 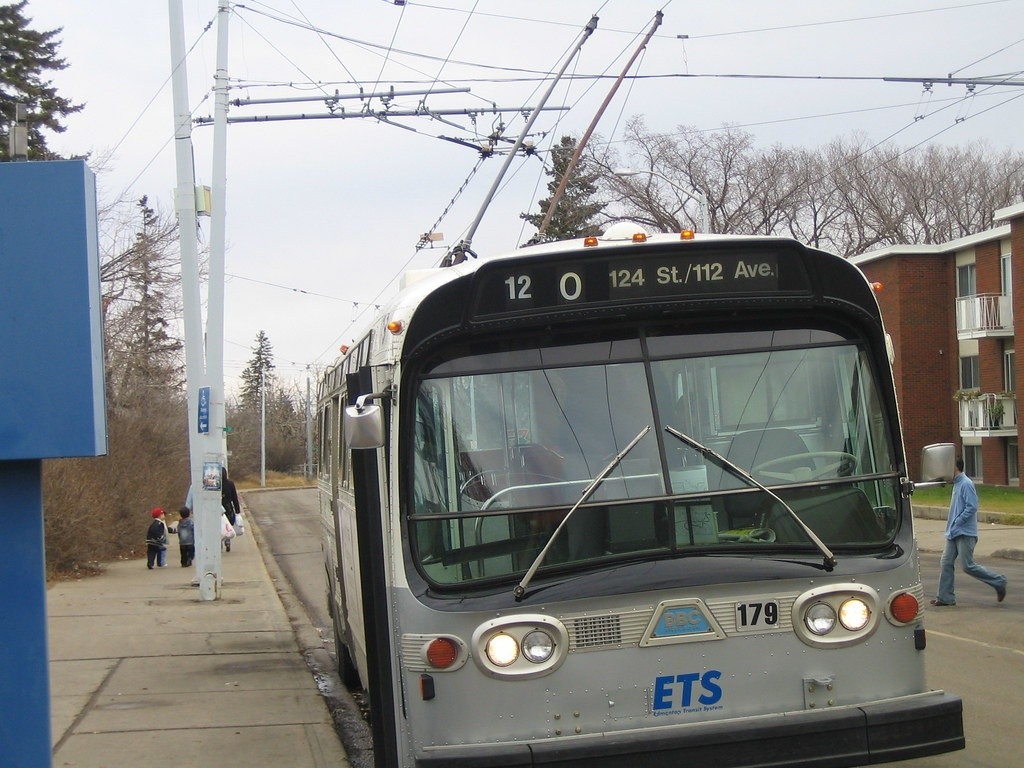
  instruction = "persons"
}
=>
[222,467,241,551]
[147,507,179,570]
[183,464,226,516]
[176,508,195,568]
[930,456,1008,606]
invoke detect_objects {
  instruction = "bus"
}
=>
[314,221,965,768]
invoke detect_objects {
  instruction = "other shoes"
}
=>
[226,545,230,552]
[998,587,1006,602]
[934,601,956,606]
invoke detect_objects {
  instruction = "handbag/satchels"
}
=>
[233,513,244,536]
[221,514,236,540]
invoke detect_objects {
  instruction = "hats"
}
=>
[152,508,164,518]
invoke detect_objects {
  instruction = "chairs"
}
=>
[719,428,819,550]
